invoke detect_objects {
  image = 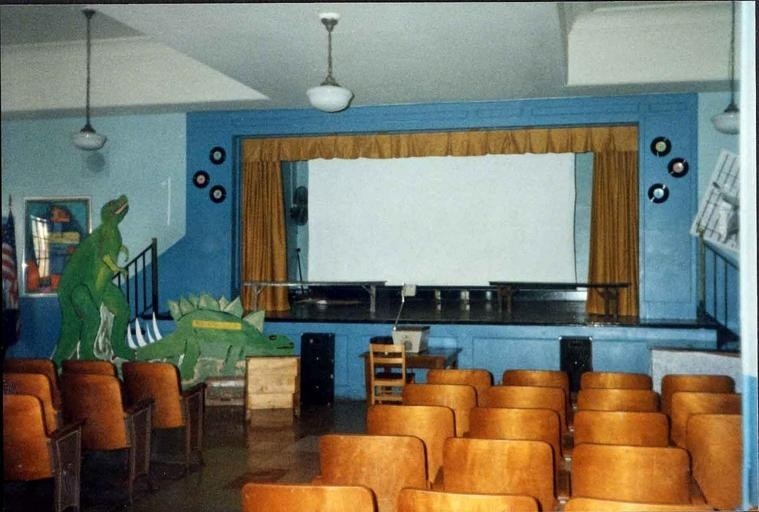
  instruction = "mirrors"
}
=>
[22,196,91,297]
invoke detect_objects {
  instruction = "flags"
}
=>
[0,195,21,362]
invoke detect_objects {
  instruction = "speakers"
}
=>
[560,336,592,393]
[302,331,335,410]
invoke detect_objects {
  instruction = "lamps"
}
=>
[711,0,741,135]
[74,8,107,151]
[307,11,355,114]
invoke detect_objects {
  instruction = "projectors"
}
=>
[392,283,431,353]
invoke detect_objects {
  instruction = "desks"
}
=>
[359,348,463,408]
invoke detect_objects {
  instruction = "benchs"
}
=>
[244,280,387,313]
[490,280,631,320]
[377,286,503,311]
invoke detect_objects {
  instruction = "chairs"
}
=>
[502,369,571,424]
[396,489,543,512]
[0,395,86,512]
[443,438,560,512]
[317,435,429,511]
[368,405,456,483]
[61,375,155,506]
[686,414,742,512]
[401,384,478,437]
[580,372,652,389]
[468,408,565,469]
[577,390,660,411]
[573,411,670,447]
[428,369,494,395]
[0,359,61,410]
[121,362,208,479]
[565,498,714,512]
[660,375,735,414]
[671,393,741,449]
[570,443,691,505]
[0,375,60,436]
[60,361,118,378]
[478,385,568,446]
[241,482,378,511]
[370,344,415,406]
